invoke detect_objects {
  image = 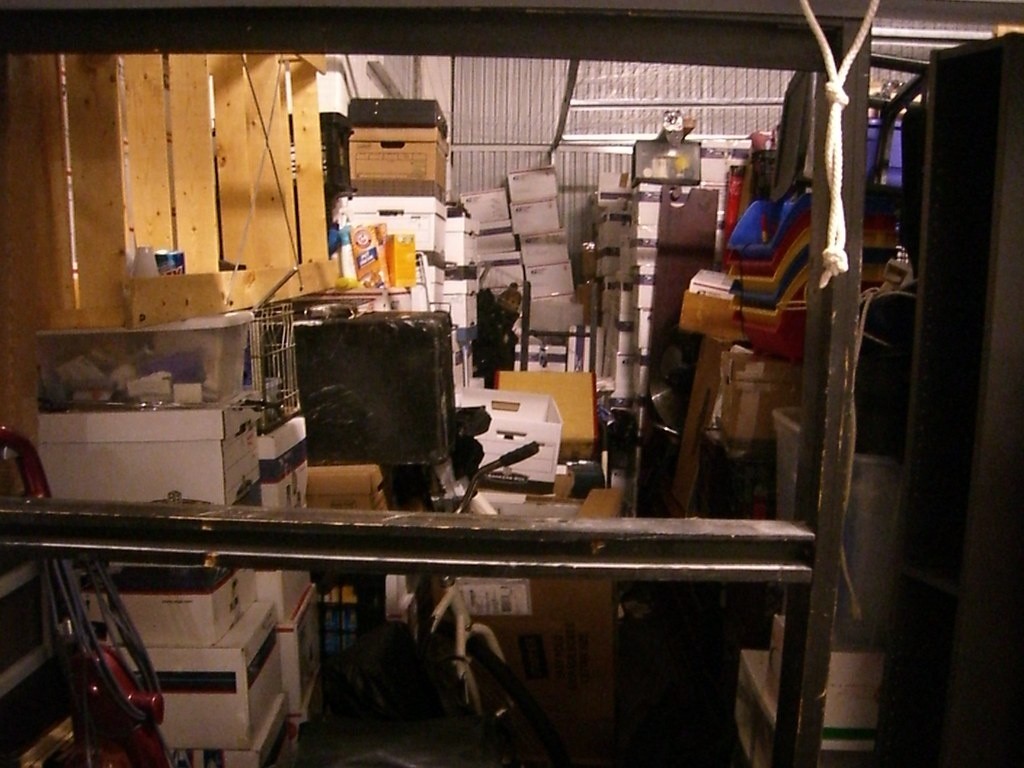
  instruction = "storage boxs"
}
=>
[36,98,918,768]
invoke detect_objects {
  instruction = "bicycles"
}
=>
[327,443,581,768]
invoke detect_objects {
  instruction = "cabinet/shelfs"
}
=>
[869,33,1024,768]
